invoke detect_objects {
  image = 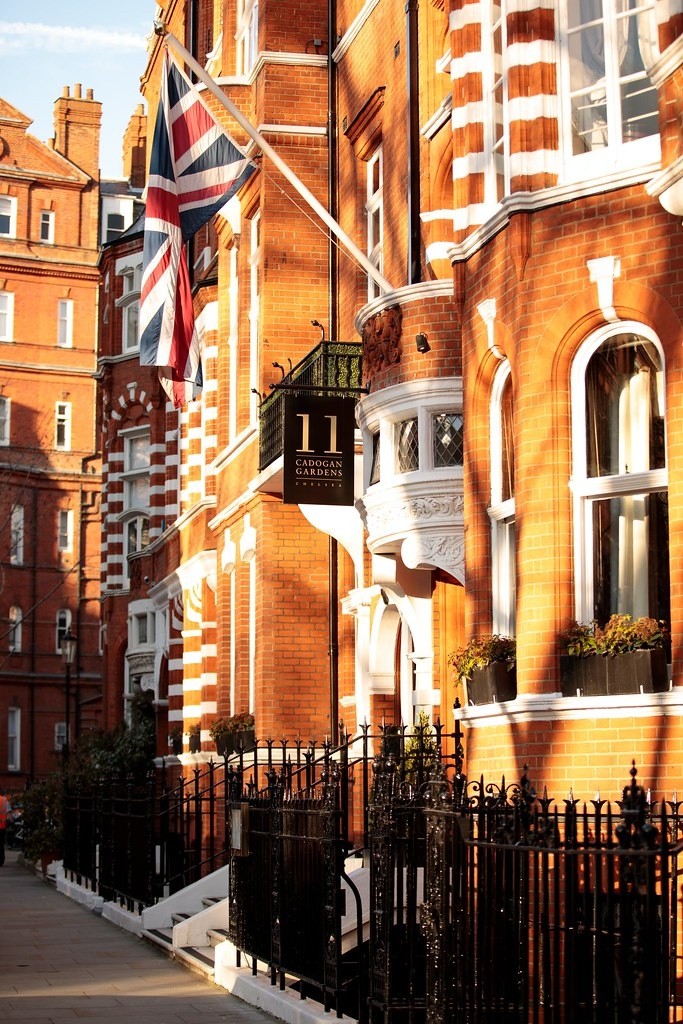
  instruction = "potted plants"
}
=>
[555,612,668,696]
[170,723,184,755]
[186,723,201,752]
[447,633,516,707]
[210,711,255,756]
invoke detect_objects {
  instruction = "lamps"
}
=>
[311,320,325,341]
[272,362,284,379]
[251,388,261,403]
[416,335,428,351]
[144,576,156,587]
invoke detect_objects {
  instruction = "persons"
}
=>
[0,788,17,866]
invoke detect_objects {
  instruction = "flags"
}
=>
[134,47,261,412]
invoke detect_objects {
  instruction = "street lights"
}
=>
[59,625,78,870]
